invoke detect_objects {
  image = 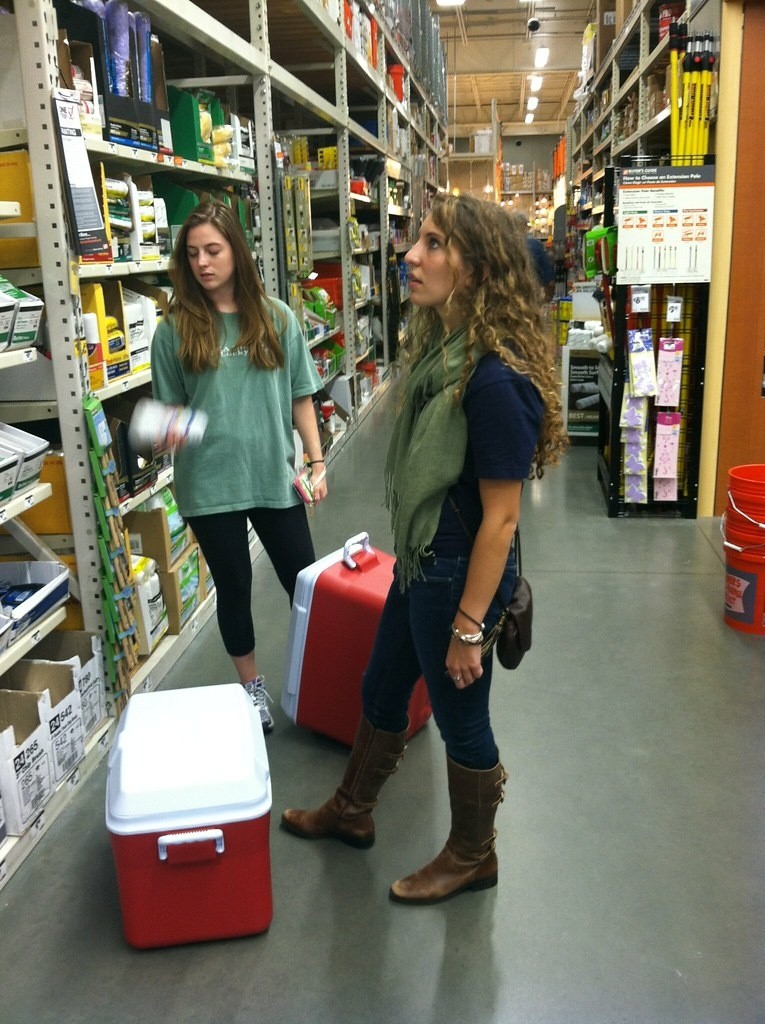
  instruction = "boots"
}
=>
[282,712,409,849]
[390,742,509,906]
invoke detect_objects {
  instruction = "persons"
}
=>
[279,191,570,906]
[512,209,557,303]
[148,199,330,734]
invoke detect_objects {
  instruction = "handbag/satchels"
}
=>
[495,575,533,670]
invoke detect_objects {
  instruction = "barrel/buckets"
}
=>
[719,462,765,635]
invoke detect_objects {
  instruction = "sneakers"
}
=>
[239,674,276,733]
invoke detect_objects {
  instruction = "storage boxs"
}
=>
[0,421,50,501]
[281,532,432,746]
[0,393,173,535]
[313,374,355,433]
[293,170,339,190]
[0,273,44,352]
[29,278,176,392]
[88,160,255,262]
[595,0,638,72]
[312,227,340,250]
[52,0,255,171]
[355,265,390,408]
[1,561,69,655]
[0,629,108,847]
[104,683,274,948]
[124,482,215,635]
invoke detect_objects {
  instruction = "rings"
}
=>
[452,673,463,682]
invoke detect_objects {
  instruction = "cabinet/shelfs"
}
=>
[1,0,449,719]
[596,155,698,519]
[565,0,703,283]
[0,347,115,891]
[498,162,554,252]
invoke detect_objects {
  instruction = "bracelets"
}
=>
[458,606,485,630]
[306,459,324,468]
[451,622,484,646]
